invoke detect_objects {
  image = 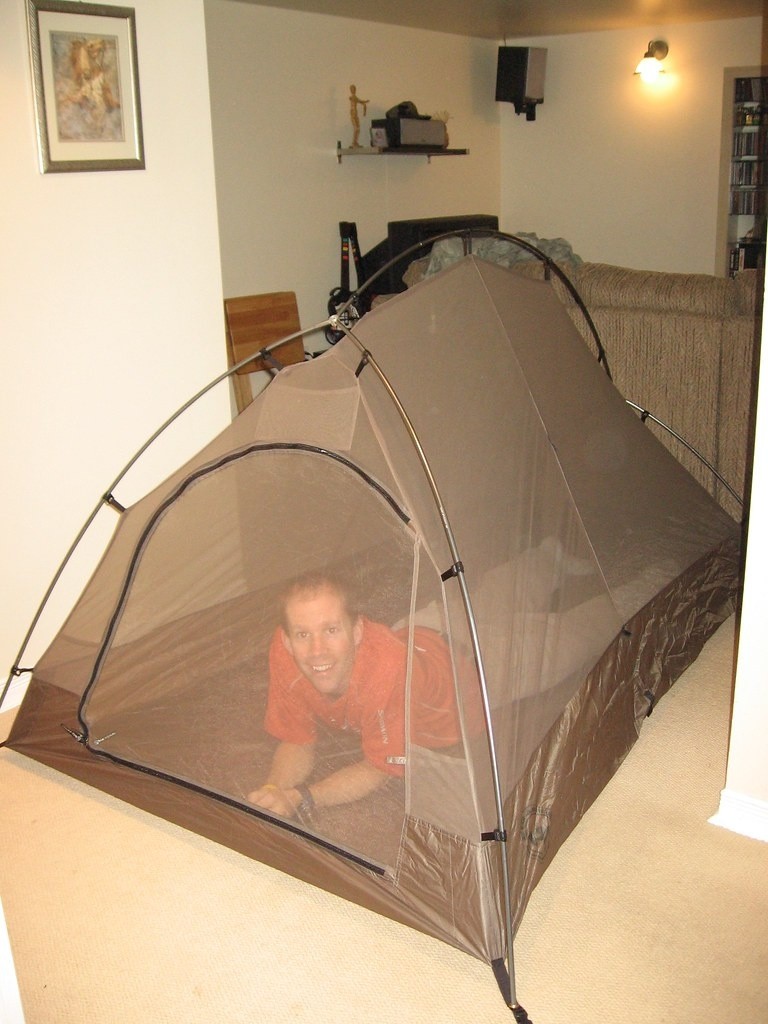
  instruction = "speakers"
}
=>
[494,46,547,103]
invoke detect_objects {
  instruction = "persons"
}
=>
[349,84,370,148]
[249,530,691,821]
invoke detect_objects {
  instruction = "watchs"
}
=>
[294,784,316,816]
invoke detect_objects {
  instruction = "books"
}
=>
[726,76,768,275]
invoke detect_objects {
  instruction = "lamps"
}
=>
[633,40,665,85]
[495,46,548,121]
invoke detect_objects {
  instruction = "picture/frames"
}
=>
[25,0,146,174]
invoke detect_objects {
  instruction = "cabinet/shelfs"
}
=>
[727,77,768,279]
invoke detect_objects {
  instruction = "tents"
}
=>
[0,231,749,1023]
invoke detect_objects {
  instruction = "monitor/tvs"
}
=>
[357,214,499,313]
